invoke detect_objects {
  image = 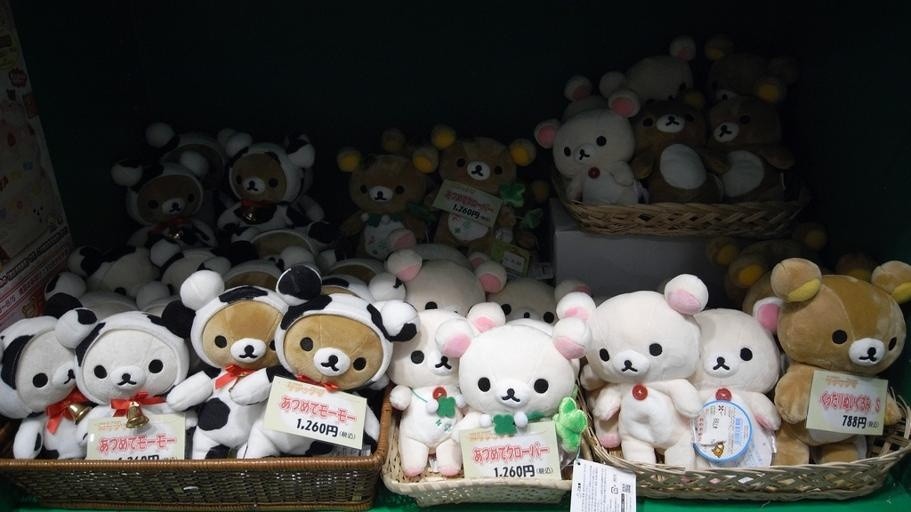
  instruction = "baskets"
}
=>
[381,415,591,507]
[548,169,806,236]
[0,379,393,510]
[577,379,911,501]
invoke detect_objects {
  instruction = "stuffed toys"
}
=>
[1,33,911,487]
[1,31,911,488]
